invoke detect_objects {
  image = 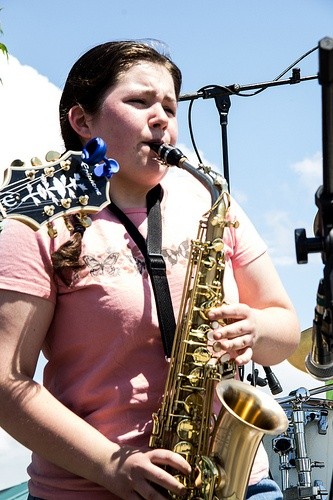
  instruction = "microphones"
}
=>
[305,277,333,381]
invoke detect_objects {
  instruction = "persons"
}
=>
[0,37,304,500]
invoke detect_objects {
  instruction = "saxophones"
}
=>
[141,142,289,500]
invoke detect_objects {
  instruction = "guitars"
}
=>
[0,136,121,232]
